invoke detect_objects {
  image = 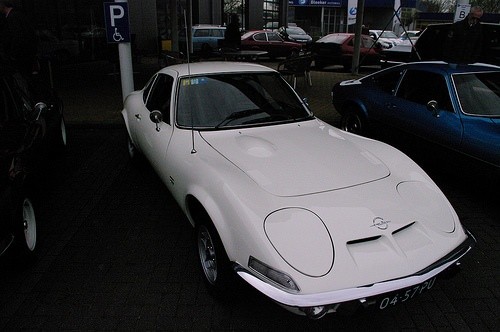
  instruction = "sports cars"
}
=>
[331,62,500,222]
[119,61,479,328]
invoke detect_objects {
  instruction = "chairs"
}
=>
[158,44,313,91]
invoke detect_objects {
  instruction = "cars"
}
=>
[263,21,281,30]
[313,33,387,72]
[238,30,301,53]
[0,77,68,261]
[366,29,402,45]
[400,31,422,38]
[279,27,313,44]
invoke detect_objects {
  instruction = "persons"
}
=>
[362,24,365,30]
[364,25,369,35]
[415,26,426,35]
[446,6,485,62]
[222,14,241,63]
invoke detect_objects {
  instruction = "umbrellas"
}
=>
[445,29,484,64]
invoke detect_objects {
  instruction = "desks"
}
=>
[222,50,269,63]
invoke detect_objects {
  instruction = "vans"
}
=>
[407,22,500,63]
[189,25,227,55]
